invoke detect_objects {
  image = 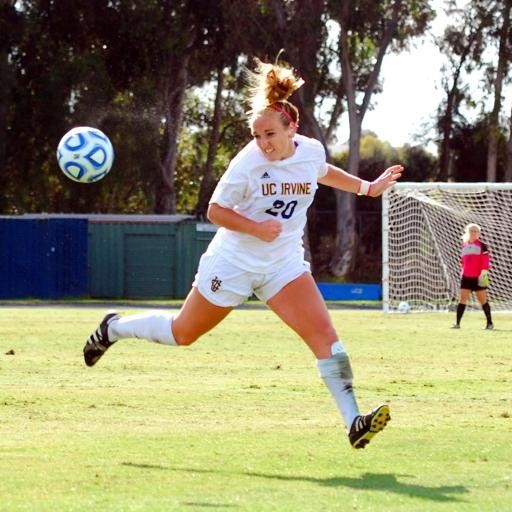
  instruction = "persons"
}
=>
[449,223,494,330]
[83,54,405,449]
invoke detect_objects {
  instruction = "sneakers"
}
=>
[449,324,461,329]
[348,404,392,450]
[83,308,121,367]
[483,323,495,332]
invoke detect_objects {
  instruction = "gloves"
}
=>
[478,269,492,287]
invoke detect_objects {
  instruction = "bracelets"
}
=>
[356,178,371,197]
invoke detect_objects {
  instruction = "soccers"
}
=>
[57,126,114,183]
[399,301,409,311]
